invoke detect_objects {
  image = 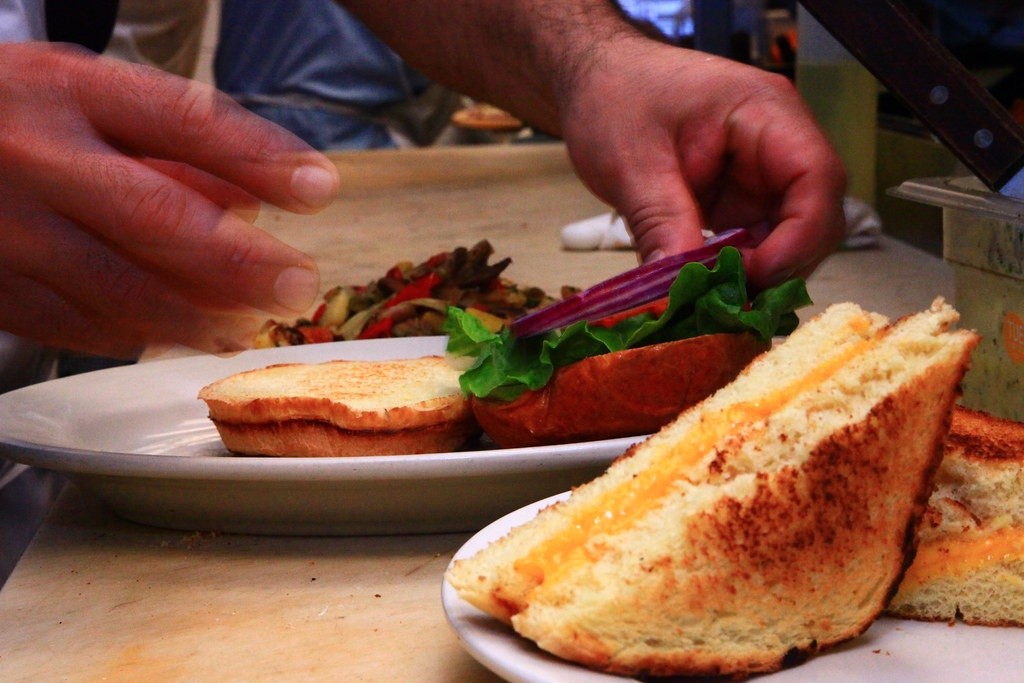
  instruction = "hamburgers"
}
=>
[445,245,813,449]
[198,358,482,454]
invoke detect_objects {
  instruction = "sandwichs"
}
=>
[445,294,982,680]
[888,404,1023,627]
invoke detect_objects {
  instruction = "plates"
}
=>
[0,335,653,532]
[440,489,1023,683]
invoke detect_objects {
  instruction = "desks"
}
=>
[0,143,1024,683]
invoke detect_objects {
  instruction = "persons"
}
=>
[212,0,462,153]
[0,0,845,587]
[105,0,211,82]
[619,0,760,63]
